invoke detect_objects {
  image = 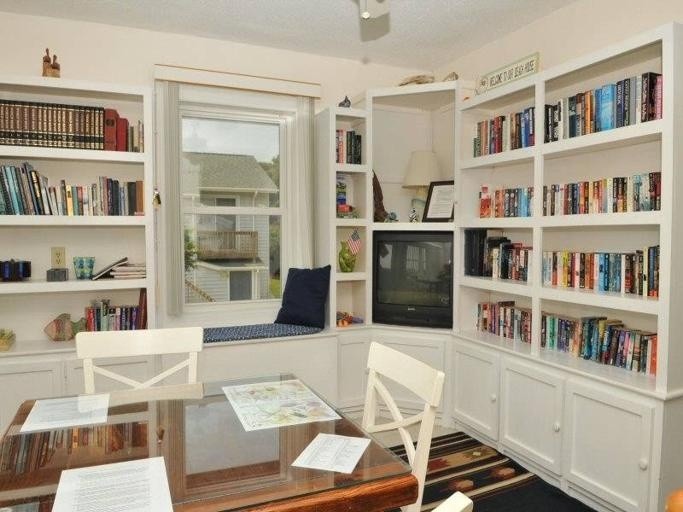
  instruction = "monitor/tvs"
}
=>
[372,232,451,328]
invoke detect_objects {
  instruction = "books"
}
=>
[541,311,656,374]
[0,98,147,332]
[1,423,146,476]
[544,72,662,143]
[465,229,533,282]
[473,107,535,157]
[479,187,534,218]
[543,246,659,297]
[336,129,361,164]
[478,301,531,343]
[543,172,661,216]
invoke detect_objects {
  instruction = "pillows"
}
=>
[275,265,331,330]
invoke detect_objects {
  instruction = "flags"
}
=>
[346,229,362,257]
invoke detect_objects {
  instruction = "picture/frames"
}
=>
[422,181,454,222]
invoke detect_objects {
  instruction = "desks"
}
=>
[1,373,421,512]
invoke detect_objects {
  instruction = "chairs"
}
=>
[361,341,472,511]
[74,325,202,392]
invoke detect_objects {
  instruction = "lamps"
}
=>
[400,149,443,222]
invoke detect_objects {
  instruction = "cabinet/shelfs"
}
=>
[500,346,566,486]
[561,356,681,511]
[173,322,339,477]
[3,356,162,435]
[367,82,456,331]
[335,329,452,429]
[453,330,532,455]
[315,106,371,327]
[460,20,683,397]
[2,78,152,356]
